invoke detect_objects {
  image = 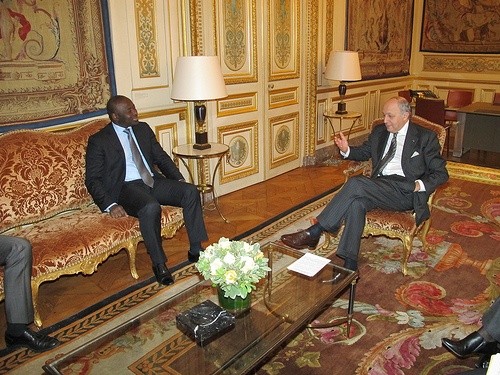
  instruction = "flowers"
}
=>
[194,237,272,300]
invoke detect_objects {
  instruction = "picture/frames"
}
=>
[0,0,117,133]
[340,0,415,83]
[420,0,500,54]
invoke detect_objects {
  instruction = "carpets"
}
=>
[0,172,500,375]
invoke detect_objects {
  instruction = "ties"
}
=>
[371,132,397,179]
[123,129,154,187]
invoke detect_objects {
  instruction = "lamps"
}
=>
[323,50,362,115]
[170,56,228,150]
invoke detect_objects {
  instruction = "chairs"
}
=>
[399,90,448,116]
[493,93,500,104]
[415,97,452,159]
[445,89,475,151]
[308,112,447,276]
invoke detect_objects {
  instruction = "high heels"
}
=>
[441,330,497,369]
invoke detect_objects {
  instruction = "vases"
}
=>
[216,283,252,319]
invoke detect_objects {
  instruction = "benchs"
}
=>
[0,117,186,331]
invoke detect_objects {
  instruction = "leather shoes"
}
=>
[152,263,174,285]
[281,229,319,250]
[188,249,205,262]
[319,268,358,284]
[4,328,60,353]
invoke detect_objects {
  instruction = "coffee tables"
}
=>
[42,240,360,375]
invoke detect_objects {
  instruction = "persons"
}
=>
[281,96,449,284]
[441,295,500,375]
[84,95,209,286]
[0,235,60,352]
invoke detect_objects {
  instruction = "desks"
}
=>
[172,142,231,225]
[452,101,500,158]
[323,111,362,165]
